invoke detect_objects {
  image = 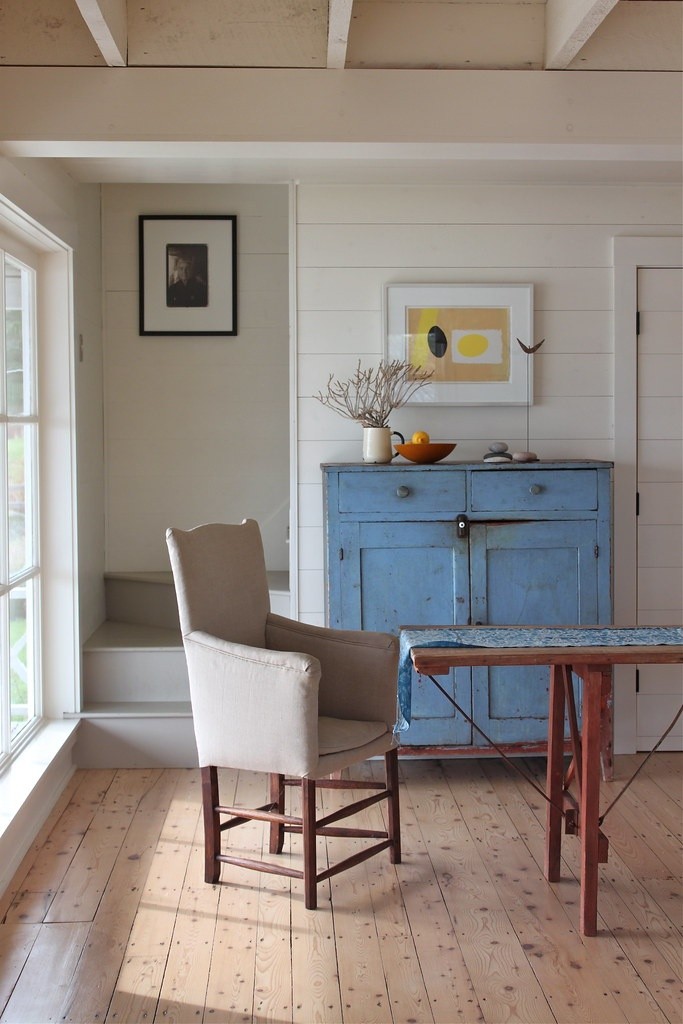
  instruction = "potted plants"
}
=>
[312,357,433,465]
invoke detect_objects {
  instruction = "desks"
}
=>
[392,624,683,937]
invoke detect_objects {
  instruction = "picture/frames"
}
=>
[138,213,238,338]
[382,283,534,407]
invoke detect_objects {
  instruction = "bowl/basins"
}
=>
[393,444,456,464]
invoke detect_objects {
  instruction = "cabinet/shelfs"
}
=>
[319,459,616,782]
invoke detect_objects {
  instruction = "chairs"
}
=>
[167,519,400,909]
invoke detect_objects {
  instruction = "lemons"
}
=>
[405,431,430,444]
